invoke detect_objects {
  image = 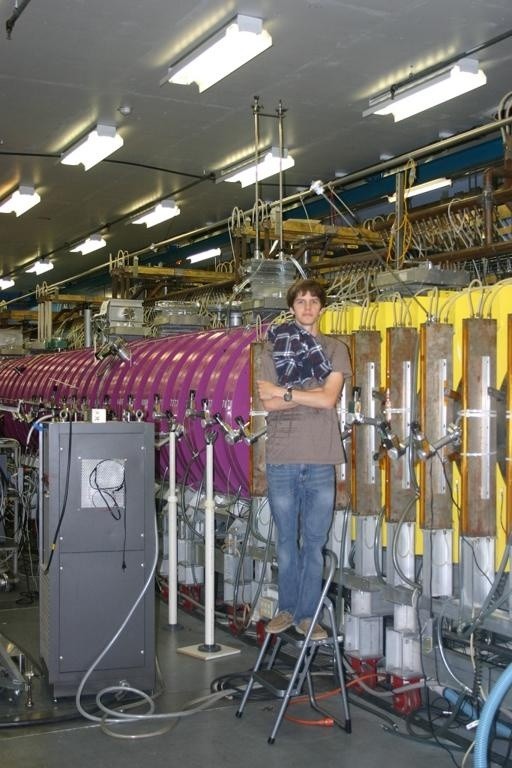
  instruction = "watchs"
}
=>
[283,386,294,402]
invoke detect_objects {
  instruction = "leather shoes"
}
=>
[264,612,328,640]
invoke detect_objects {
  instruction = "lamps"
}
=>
[186,247,222,264]
[53,122,124,172]
[159,12,272,95]
[387,176,452,204]
[124,198,181,229]
[363,58,487,123]
[0,185,42,218]
[215,146,294,188]
[0,234,107,291]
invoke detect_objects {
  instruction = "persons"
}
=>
[255,277,353,640]
